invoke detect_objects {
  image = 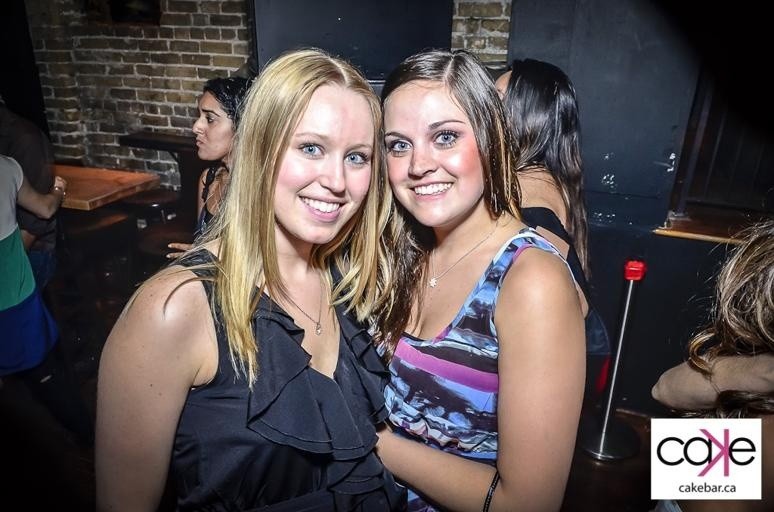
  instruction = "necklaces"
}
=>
[429,225,498,288]
[273,274,324,335]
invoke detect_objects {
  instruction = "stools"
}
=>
[46,187,198,325]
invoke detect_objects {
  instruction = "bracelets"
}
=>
[53,187,66,199]
[483,470,498,512]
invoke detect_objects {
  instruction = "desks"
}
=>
[47,161,165,211]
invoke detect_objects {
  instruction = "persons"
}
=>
[0,155,67,382]
[651,390,774,512]
[371,48,610,512]
[167,76,253,262]
[93,47,417,511]
[493,57,589,294]
[0,97,58,296]
[651,219,774,412]
